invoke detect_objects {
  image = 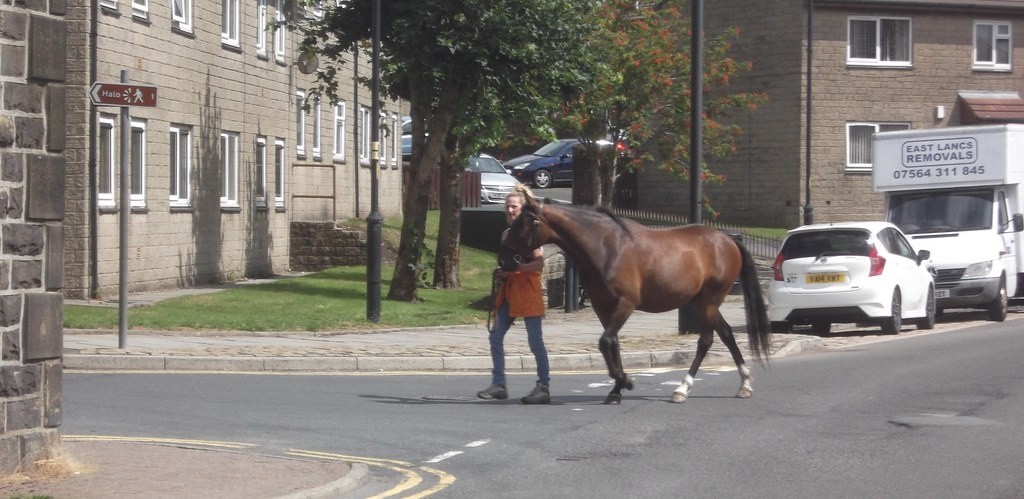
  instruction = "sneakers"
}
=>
[477,383,508,399]
[521,382,551,403]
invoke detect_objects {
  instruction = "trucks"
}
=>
[871,124,1024,323]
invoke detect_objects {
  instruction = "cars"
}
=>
[502,139,628,189]
[768,222,937,335]
[450,154,521,206]
[402,115,428,156]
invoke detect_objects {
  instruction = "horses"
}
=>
[498,185,773,405]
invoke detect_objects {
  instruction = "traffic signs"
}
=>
[88,82,157,107]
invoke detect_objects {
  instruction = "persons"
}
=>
[477,184,552,404]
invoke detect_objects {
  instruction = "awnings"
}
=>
[957,90,1024,123]
